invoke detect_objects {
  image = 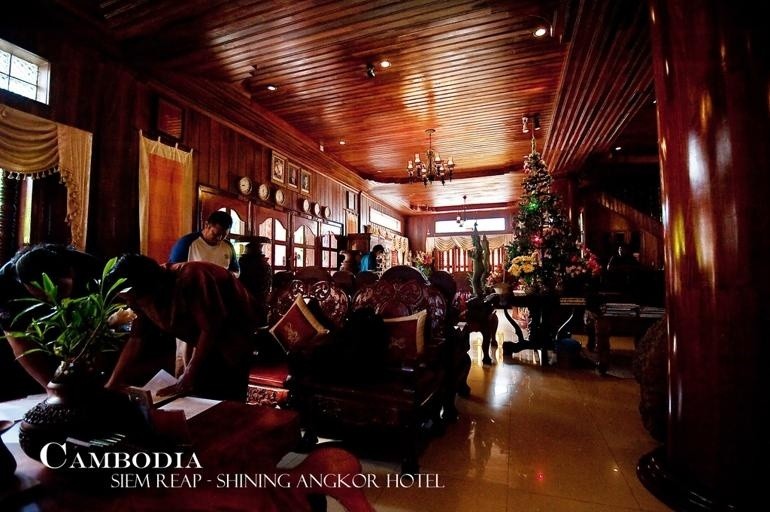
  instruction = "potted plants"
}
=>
[1,255,153,475]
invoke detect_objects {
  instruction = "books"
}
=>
[560,297,586,306]
[639,307,665,318]
[600,303,641,316]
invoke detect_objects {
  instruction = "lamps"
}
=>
[365,63,377,79]
[456,194,478,228]
[533,113,541,131]
[404,129,455,186]
[522,115,529,134]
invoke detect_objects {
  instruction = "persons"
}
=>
[603,243,640,272]
[104,254,260,404]
[0,244,106,395]
[289,168,298,185]
[359,244,385,274]
[302,174,311,190]
[169,212,241,369]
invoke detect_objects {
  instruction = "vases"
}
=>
[233,233,272,328]
[337,249,362,278]
[492,282,511,295]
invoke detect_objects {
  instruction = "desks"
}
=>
[485,291,600,368]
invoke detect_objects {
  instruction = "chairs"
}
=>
[241,279,351,447]
[331,270,358,284]
[293,265,335,285]
[380,264,473,431]
[426,269,462,324]
[352,270,380,287]
[270,270,298,285]
[297,276,467,483]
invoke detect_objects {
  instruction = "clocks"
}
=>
[238,177,253,195]
[257,183,271,202]
[274,190,287,206]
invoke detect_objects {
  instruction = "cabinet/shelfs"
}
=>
[348,229,412,273]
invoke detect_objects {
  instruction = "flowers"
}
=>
[484,263,515,288]
[509,254,537,287]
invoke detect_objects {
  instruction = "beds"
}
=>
[0,384,329,512]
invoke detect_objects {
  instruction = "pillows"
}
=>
[378,306,430,364]
[269,294,330,358]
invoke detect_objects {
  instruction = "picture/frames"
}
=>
[296,167,314,198]
[284,161,301,195]
[346,190,356,212]
[149,94,187,145]
[268,150,288,189]
